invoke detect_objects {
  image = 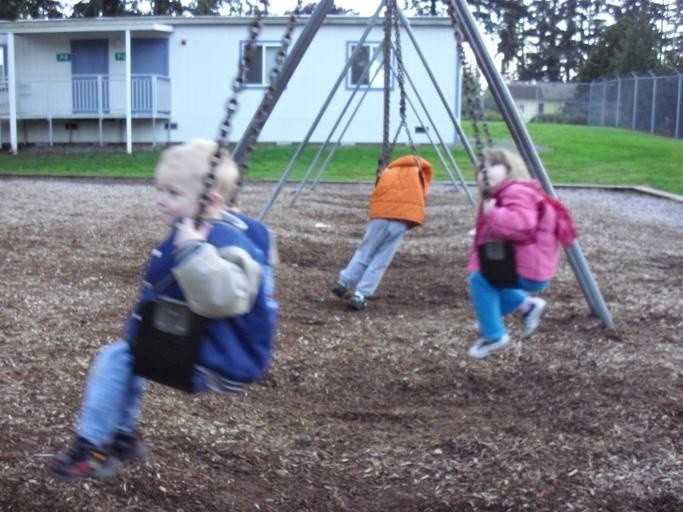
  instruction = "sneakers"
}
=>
[349,294,367,310]
[333,283,346,296]
[521,298,545,338]
[468,334,508,359]
[48,432,147,481]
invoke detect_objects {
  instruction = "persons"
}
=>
[331,155,431,311]
[466,146,577,359]
[47,138,279,480]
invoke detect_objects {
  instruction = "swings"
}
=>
[441,0,518,289]
[131,1,304,392]
[371,0,427,228]
